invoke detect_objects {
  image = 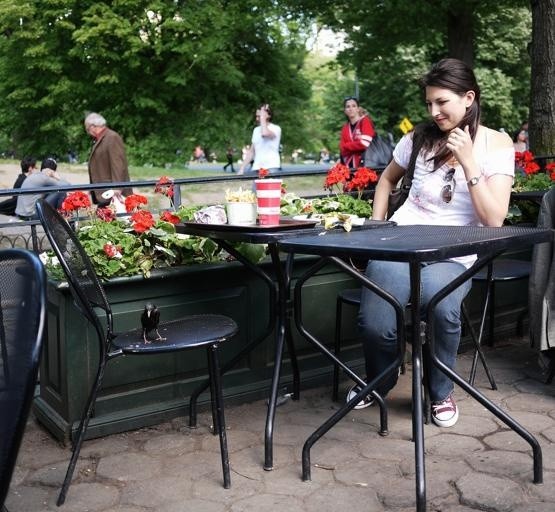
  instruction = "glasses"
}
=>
[344,96,357,100]
[442,167,455,203]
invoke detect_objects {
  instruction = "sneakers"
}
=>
[347,377,377,410]
[432,394,458,427]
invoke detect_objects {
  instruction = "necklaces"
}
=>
[445,158,458,165]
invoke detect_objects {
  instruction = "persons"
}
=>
[237,103,282,194]
[346,58,516,429]
[14,158,71,220]
[498,120,530,178]
[83,113,134,209]
[223,148,235,174]
[68,149,81,163]
[189,144,219,165]
[278,141,341,169]
[11,158,37,207]
[337,96,375,193]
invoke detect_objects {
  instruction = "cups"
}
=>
[255,178,283,227]
[225,202,257,227]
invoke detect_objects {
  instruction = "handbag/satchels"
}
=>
[364,133,395,168]
[386,178,412,221]
[103,190,130,214]
[194,206,227,226]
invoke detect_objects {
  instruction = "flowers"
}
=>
[514,149,555,191]
[44,174,222,283]
[310,161,380,217]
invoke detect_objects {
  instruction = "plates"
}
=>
[293,213,357,224]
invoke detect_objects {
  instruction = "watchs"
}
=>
[466,172,486,188]
